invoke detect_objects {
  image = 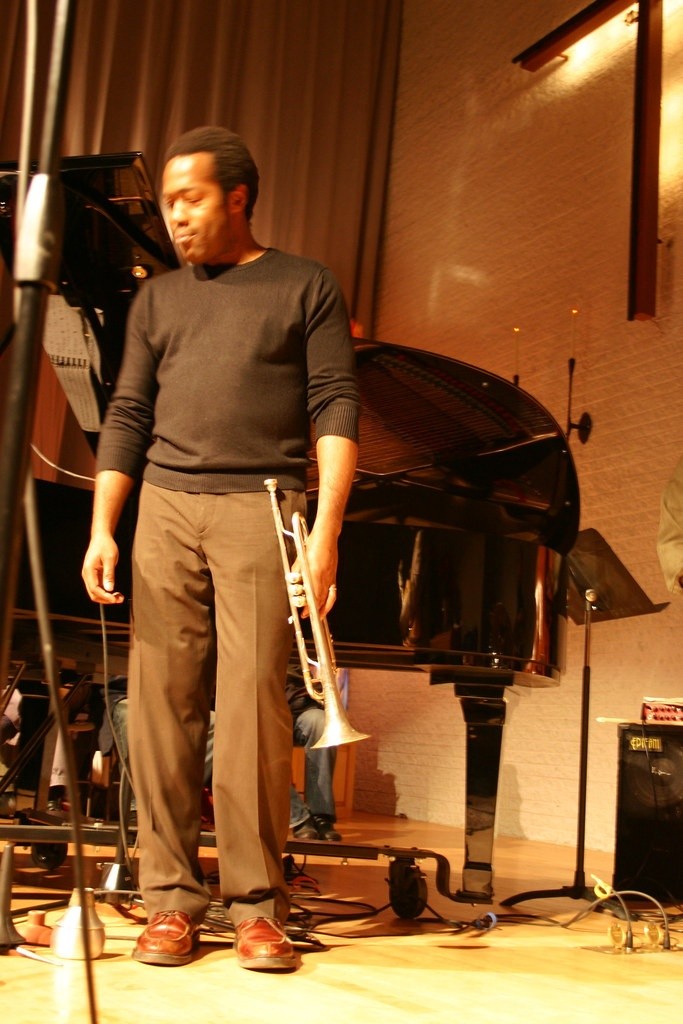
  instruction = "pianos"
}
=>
[0,147,582,901]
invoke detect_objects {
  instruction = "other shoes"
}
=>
[129,810,137,821]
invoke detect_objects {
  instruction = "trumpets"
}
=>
[264,471,369,754]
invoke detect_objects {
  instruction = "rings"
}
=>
[328,588,337,590]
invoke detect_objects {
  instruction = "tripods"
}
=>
[499,527,670,922]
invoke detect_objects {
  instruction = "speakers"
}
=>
[609,722,683,905]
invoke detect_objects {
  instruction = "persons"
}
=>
[81,125,362,970]
[284,663,342,841]
[97,675,217,786]
[0,687,70,868]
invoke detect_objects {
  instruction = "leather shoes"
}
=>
[311,813,341,840]
[32,800,68,870]
[132,910,199,964]
[293,816,321,841]
[232,917,298,968]
